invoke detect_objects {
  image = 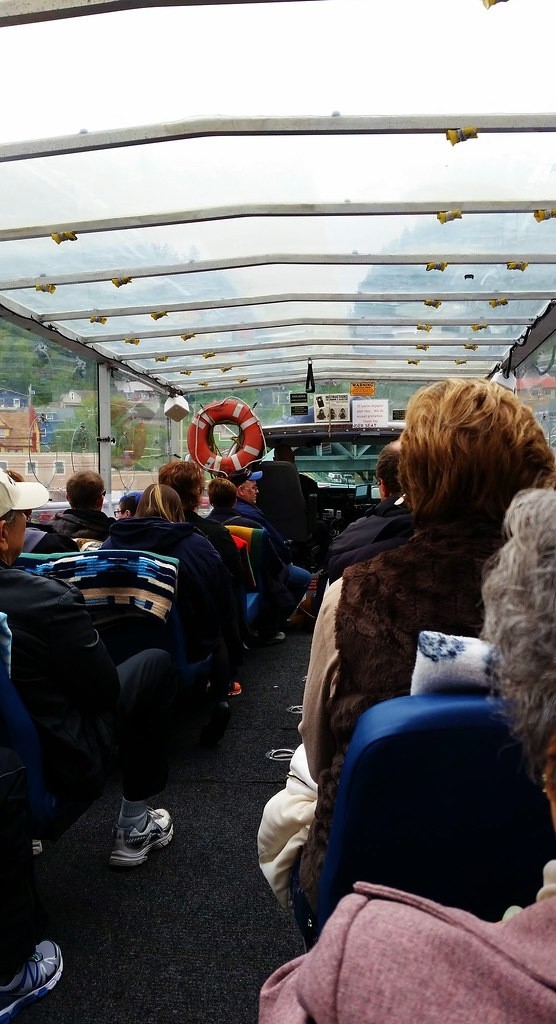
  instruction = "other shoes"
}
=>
[228,683,242,696]
[264,633,288,645]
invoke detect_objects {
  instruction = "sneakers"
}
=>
[110,807,174,866]
[32,840,44,856]
[0,940,63,1023]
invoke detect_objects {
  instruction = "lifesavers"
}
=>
[183,396,267,481]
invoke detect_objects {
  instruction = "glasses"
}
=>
[114,511,122,518]
[95,489,106,499]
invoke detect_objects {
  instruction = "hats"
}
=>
[0,469,50,519]
[228,468,264,487]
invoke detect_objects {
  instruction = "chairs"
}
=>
[224,524,284,648]
[0,661,105,937]
[289,693,556,954]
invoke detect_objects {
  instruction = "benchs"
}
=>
[13,550,217,680]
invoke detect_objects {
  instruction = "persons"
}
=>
[256,376,556,1024]
[272,444,323,520]
[0,461,312,1024]
